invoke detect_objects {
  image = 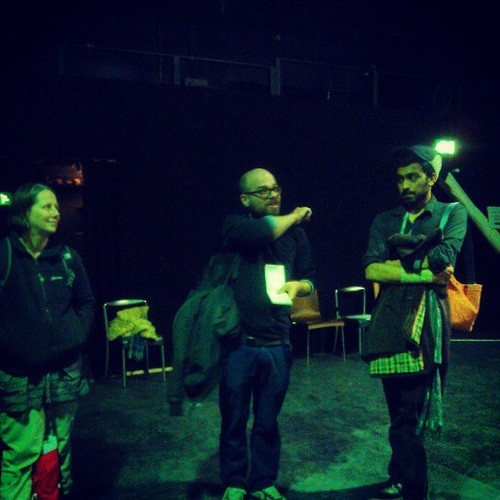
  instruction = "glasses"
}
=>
[243,185,282,201]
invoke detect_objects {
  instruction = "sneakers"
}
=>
[250,487,286,500]
[221,486,248,500]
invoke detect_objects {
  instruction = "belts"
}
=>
[234,334,289,347]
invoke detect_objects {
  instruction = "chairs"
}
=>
[102,298,167,389]
[331,286,374,362]
[288,288,347,369]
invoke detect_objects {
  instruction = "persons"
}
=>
[360,145,469,500]
[167,169,321,500]
[0,183,95,500]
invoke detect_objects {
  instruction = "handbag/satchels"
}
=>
[446,274,483,335]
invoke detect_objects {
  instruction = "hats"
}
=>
[391,144,443,179]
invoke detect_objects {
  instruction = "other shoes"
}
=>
[379,482,417,500]
